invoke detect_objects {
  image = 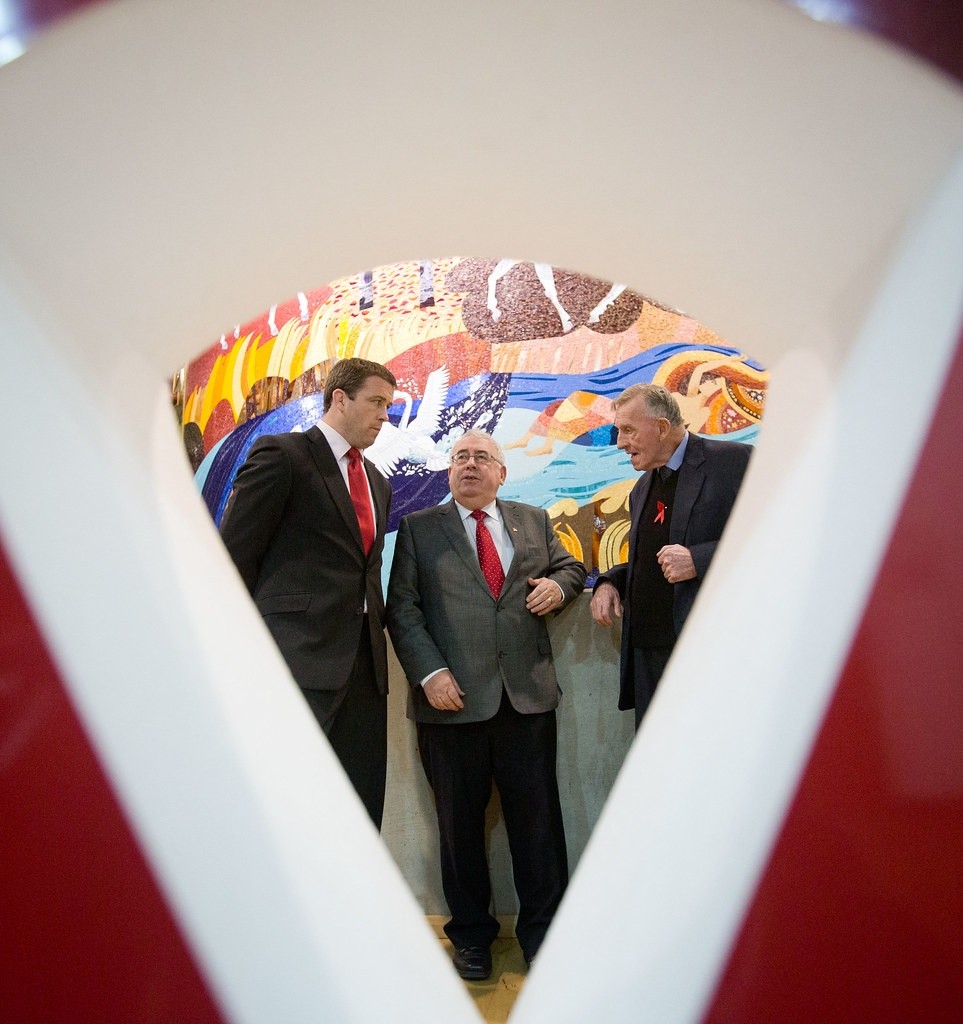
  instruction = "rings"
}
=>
[548,598,553,603]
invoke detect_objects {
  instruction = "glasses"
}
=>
[450,453,503,466]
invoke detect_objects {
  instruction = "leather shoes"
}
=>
[453,945,492,979]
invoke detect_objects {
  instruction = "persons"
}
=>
[215,356,397,831]
[590,384,755,733]
[381,432,588,982]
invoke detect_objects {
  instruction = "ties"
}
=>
[346,448,375,559]
[659,465,672,482]
[470,509,506,602]
